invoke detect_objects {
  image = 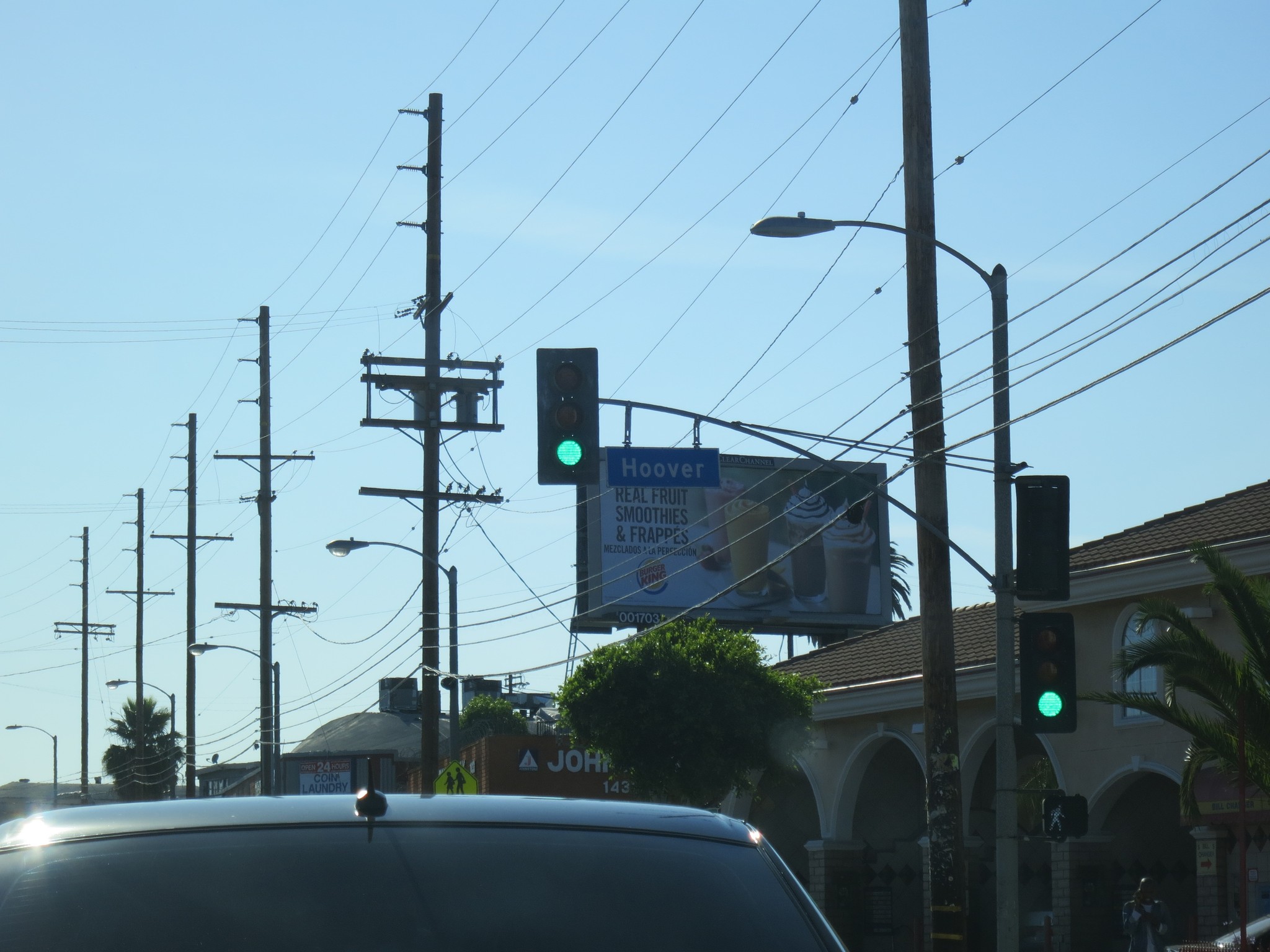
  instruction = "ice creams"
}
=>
[785,485,874,546]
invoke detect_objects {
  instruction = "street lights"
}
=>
[105,677,177,801]
[324,536,463,795]
[748,212,1020,952]
[186,642,283,795]
[5,724,58,810]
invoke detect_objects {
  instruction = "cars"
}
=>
[1163,913,1270,952]
[0,795,848,952]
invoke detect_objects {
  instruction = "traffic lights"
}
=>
[1040,794,1088,839]
[535,347,600,487]
[1020,610,1080,733]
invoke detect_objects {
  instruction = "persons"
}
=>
[1123,877,1173,952]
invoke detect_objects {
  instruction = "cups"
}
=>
[705,480,743,570]
[725,503,771,597]
[783,515,827,604]
[822,534,876,615]
